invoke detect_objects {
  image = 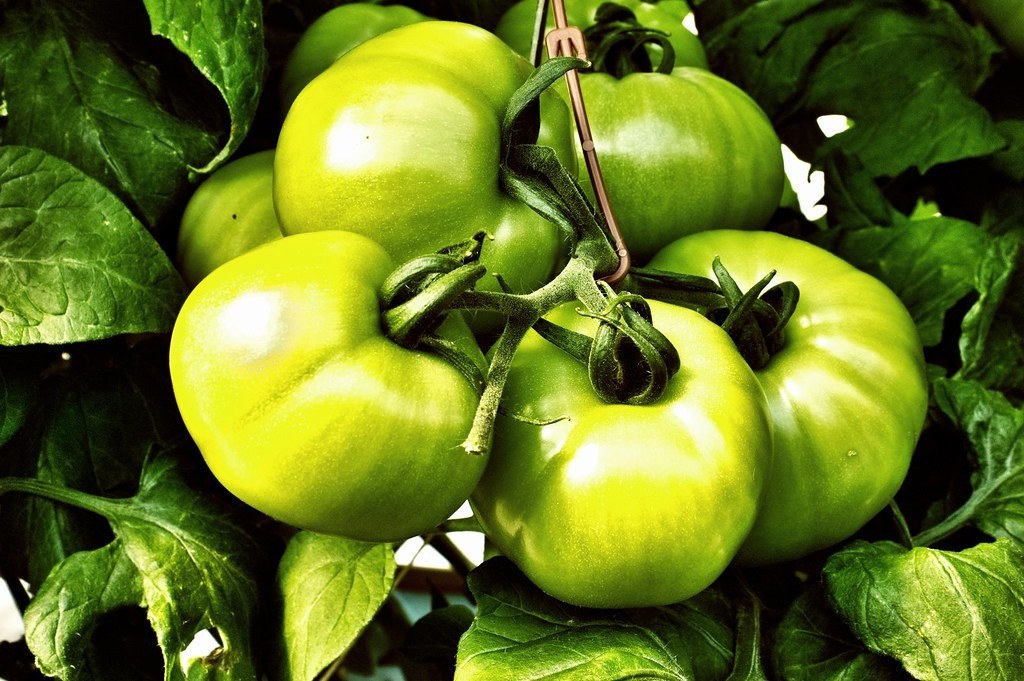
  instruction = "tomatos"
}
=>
[166,0,929,613]
